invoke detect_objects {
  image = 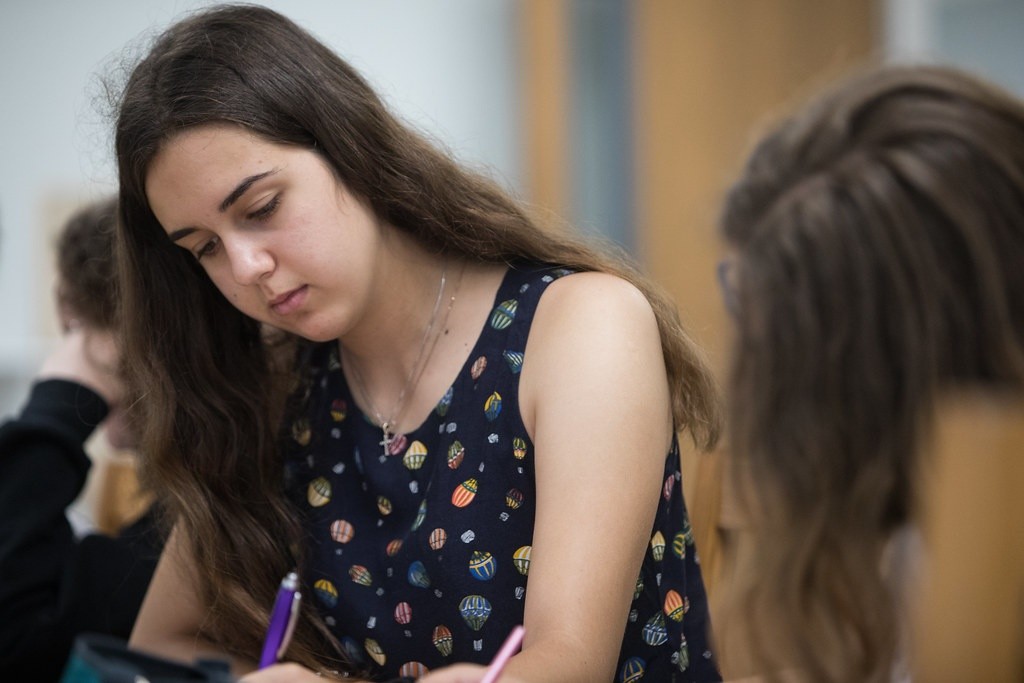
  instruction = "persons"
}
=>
[706,58,1024,683]
[0,194,180,683]
[93,5,719,682]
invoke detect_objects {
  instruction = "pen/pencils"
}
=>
[479,624,526,683]
[257,573,302,671]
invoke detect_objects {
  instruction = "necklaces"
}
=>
[345,254,461,456]
